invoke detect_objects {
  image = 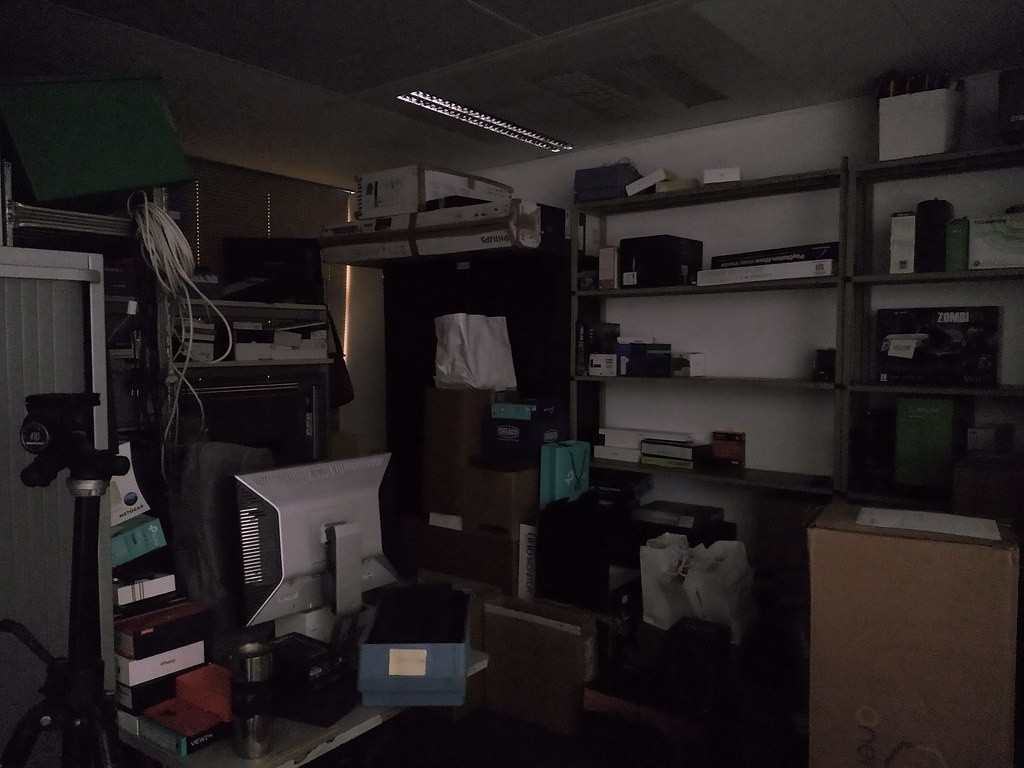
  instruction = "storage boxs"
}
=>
[807,503,1020,768]
[423,385,599,738]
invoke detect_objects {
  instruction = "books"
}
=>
[592,427,746,471]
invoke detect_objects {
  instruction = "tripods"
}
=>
[0,393,133,768]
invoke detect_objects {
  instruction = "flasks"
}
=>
[230,641,272,760]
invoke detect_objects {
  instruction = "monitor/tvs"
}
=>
[223,452,397,631]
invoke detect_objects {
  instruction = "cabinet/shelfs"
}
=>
[569,142,1023,522]
